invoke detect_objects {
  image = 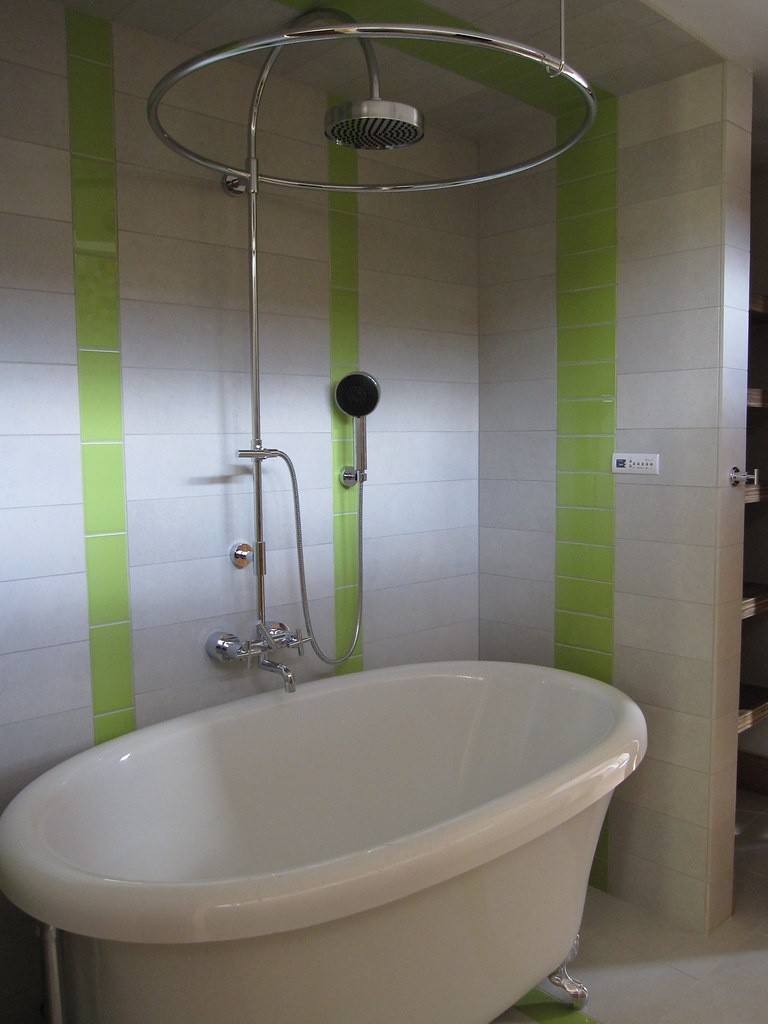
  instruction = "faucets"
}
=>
[261,659,297,693]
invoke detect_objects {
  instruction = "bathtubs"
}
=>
[0,659,650,1023]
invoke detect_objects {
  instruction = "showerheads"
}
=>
[333,371,380,483]
[323,100,424,151]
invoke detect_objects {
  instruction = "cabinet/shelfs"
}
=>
[737,298,768,732]
